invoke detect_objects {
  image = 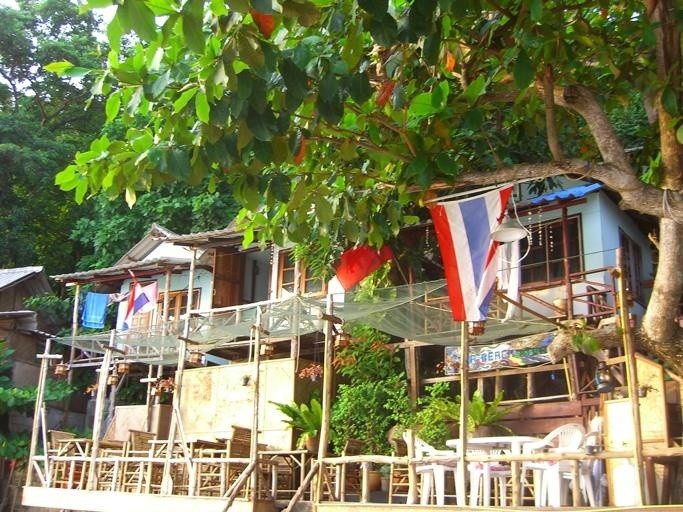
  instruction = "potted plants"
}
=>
[85,383,98,397]
[267,398,325,457]
[455,389,531,437]
[155,377,178,394]
[330,371,413,493]
[298,363,323,384]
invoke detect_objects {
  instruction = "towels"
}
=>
[80,290,109,330]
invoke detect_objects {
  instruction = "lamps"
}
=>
[593,358,614,395]
[333,331,350,350]
[116,362,130,375]
[466,318,486,336]
[55,363,68,378]
[487,206,527,246]
[258,339,273,357]
[188,351,205,366]
[107,374,119,385]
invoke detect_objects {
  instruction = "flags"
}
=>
[120,269,158,331]
[326,239,398,294]
[423,184,516,325]
